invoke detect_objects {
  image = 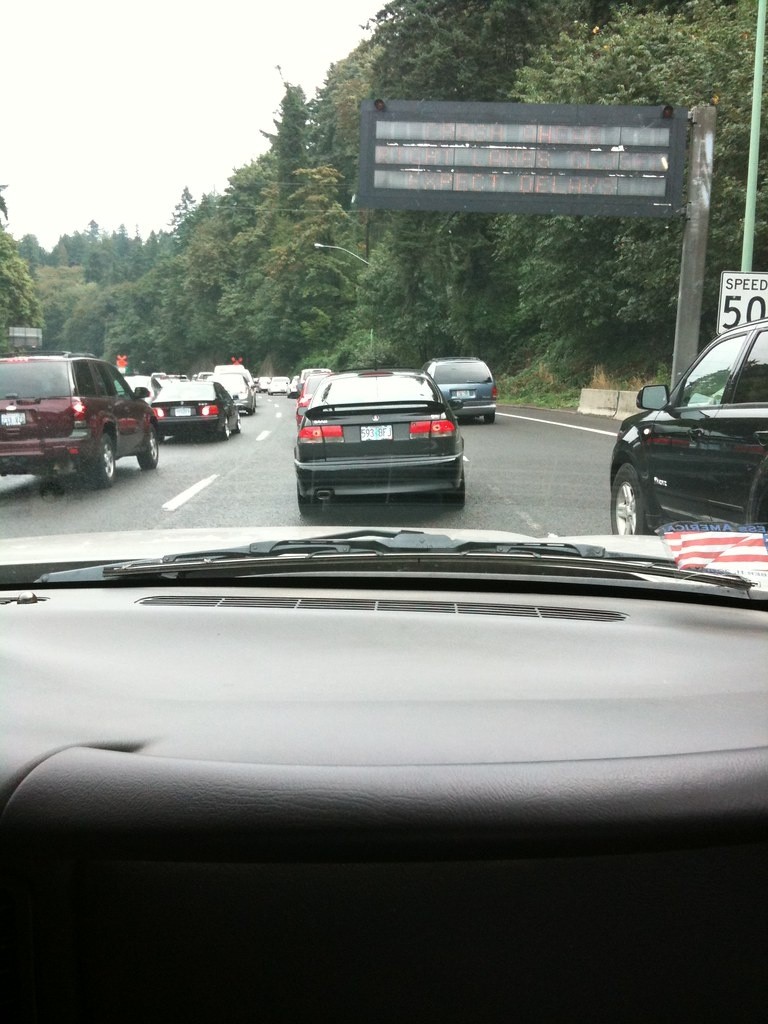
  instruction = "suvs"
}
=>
[608,315,768,538]
[0,349,162,489]
[422,355,497,425]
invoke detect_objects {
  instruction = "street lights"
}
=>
[314,242,376,351]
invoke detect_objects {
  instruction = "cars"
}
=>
[291,367,466,515]
[113,362,341,440]
[150,378,241,441]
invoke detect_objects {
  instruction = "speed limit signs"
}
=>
[717,269,768,336]
